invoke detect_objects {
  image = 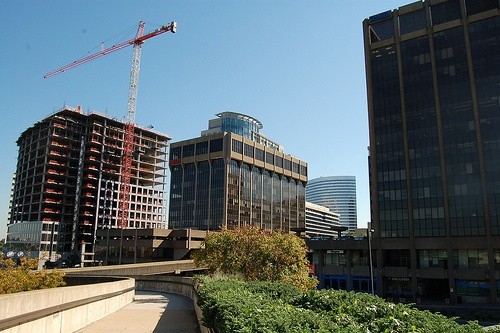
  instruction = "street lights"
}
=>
[367,229,376,297]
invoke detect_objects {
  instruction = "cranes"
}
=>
[41,20,177,230]
